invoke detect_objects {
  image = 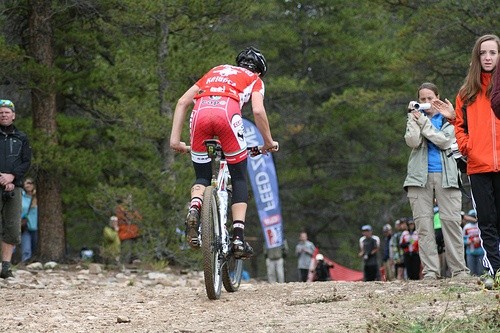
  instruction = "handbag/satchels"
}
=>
[21,217,27,233]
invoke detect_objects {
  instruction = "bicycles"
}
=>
[173,144,279,301]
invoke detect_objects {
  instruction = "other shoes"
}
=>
[0,269,14,278]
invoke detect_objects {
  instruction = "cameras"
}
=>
[2,191,15,201]
[414,103,431,111]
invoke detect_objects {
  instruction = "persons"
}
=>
[265,82,486,284]
[170,46,280,260]
[103,196,142,264]
[0,100,37,278]
[455,35,499,290]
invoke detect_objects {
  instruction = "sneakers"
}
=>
[187,207,201,250]
[231,239,256,257]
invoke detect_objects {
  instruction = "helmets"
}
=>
[362,225,372,231]
[0,99,15,112]
[316,254,323,261]
[237,46,268,77]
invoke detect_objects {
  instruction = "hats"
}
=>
[401,218,407,223]
[433,207,439,214]
[408,220,414,224]
[468,210,476,216]
[383,224,391,232]
